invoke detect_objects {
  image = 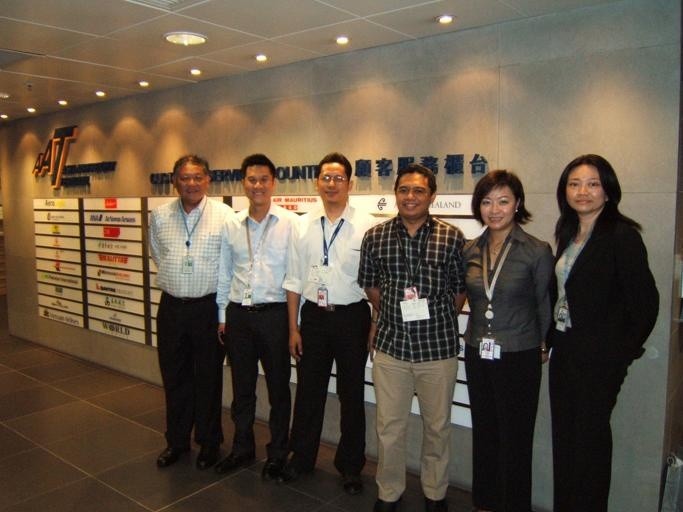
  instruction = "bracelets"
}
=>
[541,348,547,351]
[370,319,377,323]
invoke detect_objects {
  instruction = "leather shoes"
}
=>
[261,458,286,481]
[197,445,223,467]
[344,474,362,494]
[281,462,313,479]
[157,444,190,466]
[425,496,448,512]
[373,496,402,512]
[215,452,255,473]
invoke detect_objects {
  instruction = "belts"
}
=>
[236,303,285,310]
[163,292,215,304]
[306,300,366,312]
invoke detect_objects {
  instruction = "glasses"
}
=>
[321,176,348,182]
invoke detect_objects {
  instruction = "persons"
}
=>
[462,170,552,511]
[147,154,234,468]
[548,155,659,512]
[557,311,565,323]
[215,153,304,481]
[278,153,382,496]
[482,343,488,351]
[358,164,466,511]
